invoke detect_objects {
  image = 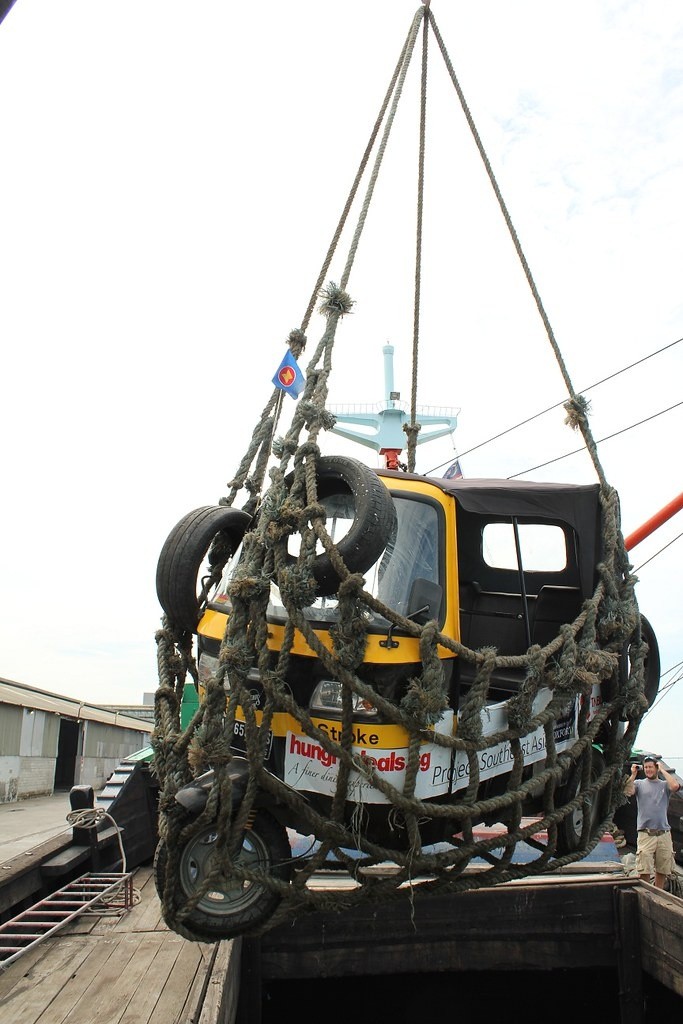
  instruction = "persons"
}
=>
[624,755,681,890]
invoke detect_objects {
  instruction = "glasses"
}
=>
[644,755,655,761]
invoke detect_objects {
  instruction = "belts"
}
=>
[640,827,668,836]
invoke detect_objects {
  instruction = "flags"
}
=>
[270,348,307,400]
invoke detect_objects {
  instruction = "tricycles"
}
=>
[146,468,630,940]
